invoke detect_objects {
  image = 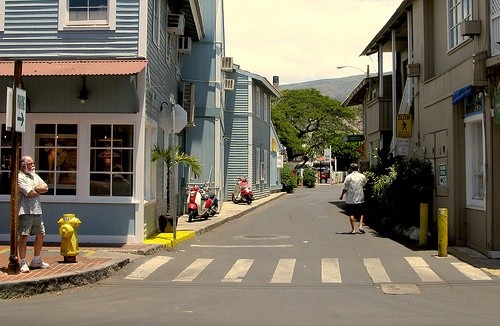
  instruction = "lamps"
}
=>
[76,76,89,104]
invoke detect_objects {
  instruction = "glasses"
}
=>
[23,162,35,165]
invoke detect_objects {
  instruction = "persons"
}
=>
[339,163,367,233]
[16,156,50,273]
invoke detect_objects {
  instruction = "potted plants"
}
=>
[281,164,301,193]
[151,144,202,233]
[303,168,316,188]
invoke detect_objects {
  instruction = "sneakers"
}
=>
[19,263,30,273]
[30,259,49,268]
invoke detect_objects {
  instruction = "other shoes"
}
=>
[359,227,365,233]
[350,231,355,233]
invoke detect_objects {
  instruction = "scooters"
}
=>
[232,177,254,205]
[186,181,218,222]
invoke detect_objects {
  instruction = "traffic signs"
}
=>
[16,88,27,133]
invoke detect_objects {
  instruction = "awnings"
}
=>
[0,60,148,76]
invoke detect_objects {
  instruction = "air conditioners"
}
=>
[178,37,192,54]
[166,13,185,35]
[222,56,233,72]
[181,83,196,128]
[225,79,235,90]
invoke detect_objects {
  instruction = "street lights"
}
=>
[337,64,370,78]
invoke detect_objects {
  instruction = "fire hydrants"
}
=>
[57,214,83,262]
